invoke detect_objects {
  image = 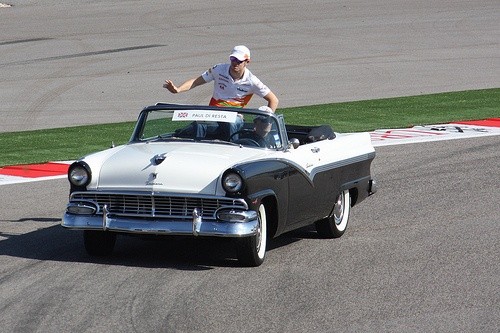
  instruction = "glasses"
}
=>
[255,117,275,123]
[230,56,247,63]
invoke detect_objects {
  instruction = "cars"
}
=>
[61,104,376,266]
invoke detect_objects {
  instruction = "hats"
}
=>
[229,45,250,61]
[253,106,275,121]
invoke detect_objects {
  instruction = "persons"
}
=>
[163,45,278,123]
[252,104,285,152]
